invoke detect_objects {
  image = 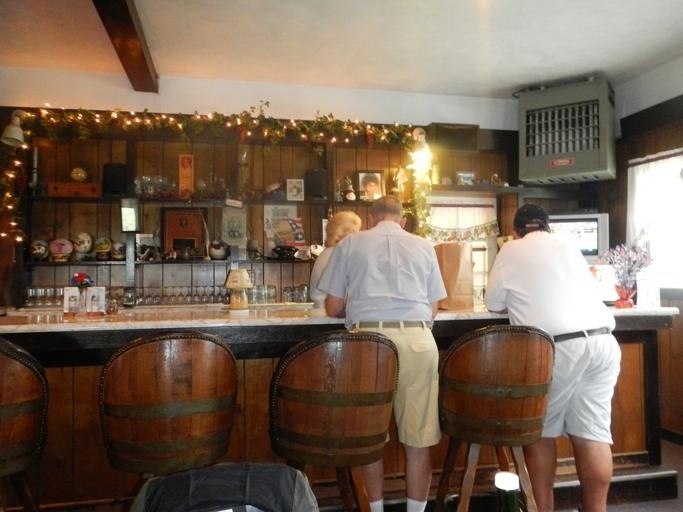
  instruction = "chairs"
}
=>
[435,325,558,512]
[98,327,238,512]
[0,339,50,511]
[267,330,399,511]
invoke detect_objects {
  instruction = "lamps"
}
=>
[224,269,253,309]
[1,115,25,148]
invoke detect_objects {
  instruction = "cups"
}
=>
[293,288,301,300]
[44,287,56,307]
[54,287,65,306]
[258,285,269,304]
[250,285,259,304]
[301,284,309,301]
[282,286,291,301]
[25,287,36,306]
[33,287,46,305]
[269,284,278,304]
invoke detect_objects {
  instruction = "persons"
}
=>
[316,195,449,512]
[309,210,362,308]
[360,174,380,201]
[482,204,622,512]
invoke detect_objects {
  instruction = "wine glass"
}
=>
[209,286,217,301]
[216,286,224,302]
[152,286,162,305]
[193,285,201,303]
[144,286,154,304]
[162,285,170,304]
[135,286,143,307]
[169,285,178,304]
[178,285,186,304]
[202,285,208,303]
[224,289,230,304]
[186,285,193,303]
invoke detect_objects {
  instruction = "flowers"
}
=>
[601,232,653,282]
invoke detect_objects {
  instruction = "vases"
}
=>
[615,284,636,309]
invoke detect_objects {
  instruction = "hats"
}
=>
[513,204,549,231]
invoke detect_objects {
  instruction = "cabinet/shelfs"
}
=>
[14,130,414,302]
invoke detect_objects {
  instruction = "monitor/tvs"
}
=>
[549,214,610,266]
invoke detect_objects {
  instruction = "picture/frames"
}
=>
[286,179,305,201]
[179,154,194,196]
[356,168,387,201]
[159,206,209,262]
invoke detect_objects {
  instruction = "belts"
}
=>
[352,320,432,329]
[554,327,610,342]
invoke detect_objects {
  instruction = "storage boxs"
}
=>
[47,182,102,198]
[434,242,475,311]
[428,122,481,154]
